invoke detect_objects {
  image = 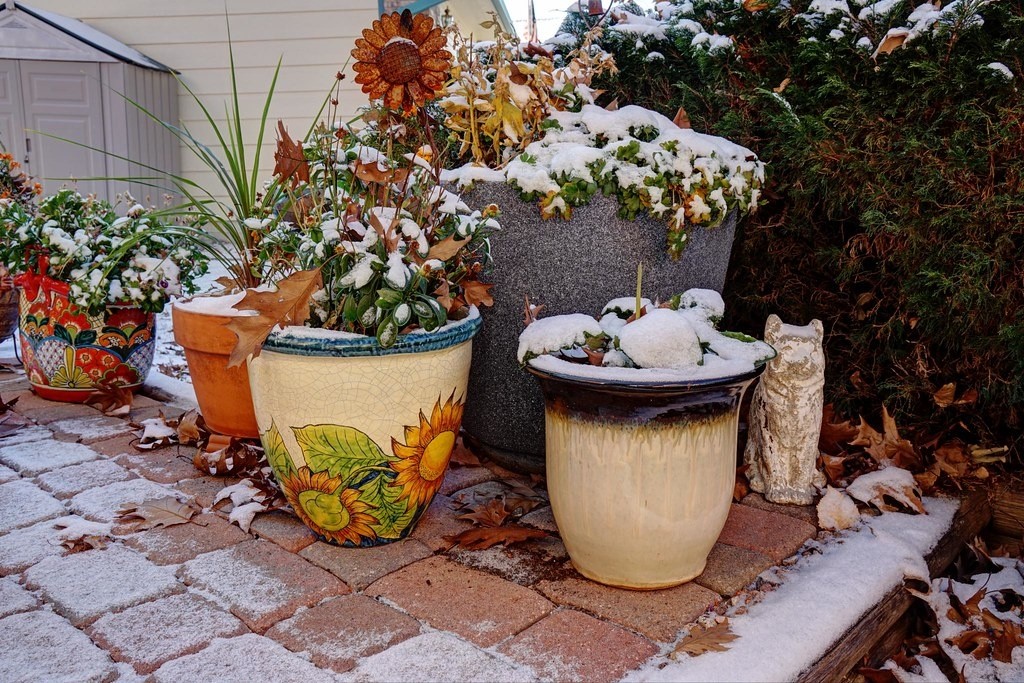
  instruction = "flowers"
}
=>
[1,154,41,279]
[0,172,213,315]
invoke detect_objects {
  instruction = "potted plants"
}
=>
[350,0,768,478]
[23,0,354,439]
[221,69,501,547]
[512,260,779,593]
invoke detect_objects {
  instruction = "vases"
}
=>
[14,239,161,401]
[0,264,23,343]
